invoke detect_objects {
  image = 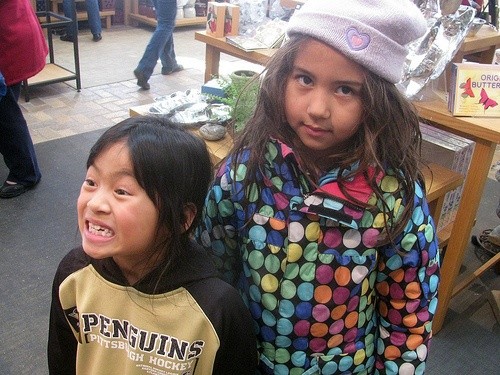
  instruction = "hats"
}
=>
[287,0,427,84]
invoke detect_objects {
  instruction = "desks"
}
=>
[194,23,500,335]
[129,94,479,269]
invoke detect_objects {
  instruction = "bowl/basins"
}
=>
[468,18,487,37]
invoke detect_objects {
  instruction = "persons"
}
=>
[200,0,444,375]
[47,114,263,375]
[55,0,104,42]
[1,0,51,200]
[134,0,186,90]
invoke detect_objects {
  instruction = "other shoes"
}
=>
[0,181,32,198]
[93,33,102,42]
[133,68,150,90]
[60,32,74,41]
[161,63,184,75]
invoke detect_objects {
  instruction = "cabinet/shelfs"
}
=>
[19,0,232,103]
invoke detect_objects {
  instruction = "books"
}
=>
[205,1,226,38]
[224,3,240,37]
[448,62,500,118]
[226,35,268,53]
[246,17,291,50]
